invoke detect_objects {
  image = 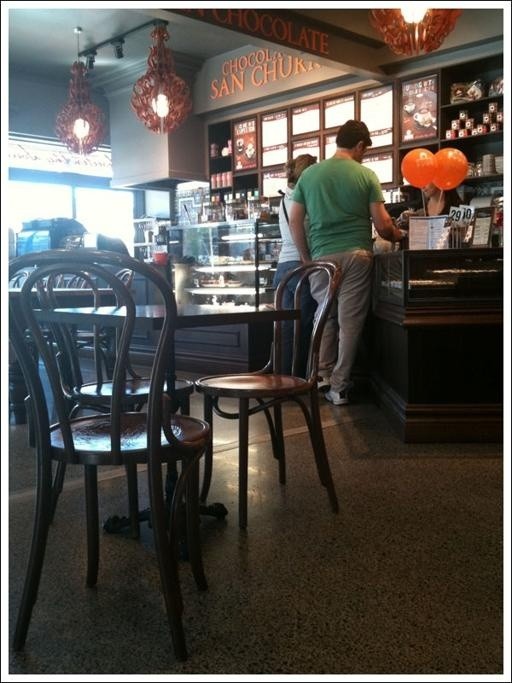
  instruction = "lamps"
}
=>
[78,19,169,71]
[52,25,109,156]
[129,21,195,137]
[366,9,465,60]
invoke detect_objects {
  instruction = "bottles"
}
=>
[210,142,219,158]
[227,139,232,155]
[200,190,260,222]
[210,171,233,188]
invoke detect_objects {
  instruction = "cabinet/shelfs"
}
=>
[207,119,233,202]
[165,218,282,313]
[370,244,503,445]
[439,53,504,215]
[14,216,89,259]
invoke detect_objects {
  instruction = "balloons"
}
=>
[400,149,439,191]
[433,148,469,191]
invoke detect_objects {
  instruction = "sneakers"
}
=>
[317,378,349,406]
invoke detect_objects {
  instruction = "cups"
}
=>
[248,195,271,225]
[154,251,169,265]
[404,102,415,111]
[413,108,433,123]
[144,231,152,242]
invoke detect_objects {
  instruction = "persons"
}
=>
[272,153,318,378]
[287,119,401,405]
[419,184,453,216]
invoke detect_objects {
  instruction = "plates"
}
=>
[482,153,503,176]
[199,283,241,288]
[423,116,437,128]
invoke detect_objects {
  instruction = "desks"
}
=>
[34,303,303,530]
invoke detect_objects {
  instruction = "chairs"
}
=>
[9,266,134,422]
[34,251,197,540]
[195,261,340,529]
[9,251,211,662]
